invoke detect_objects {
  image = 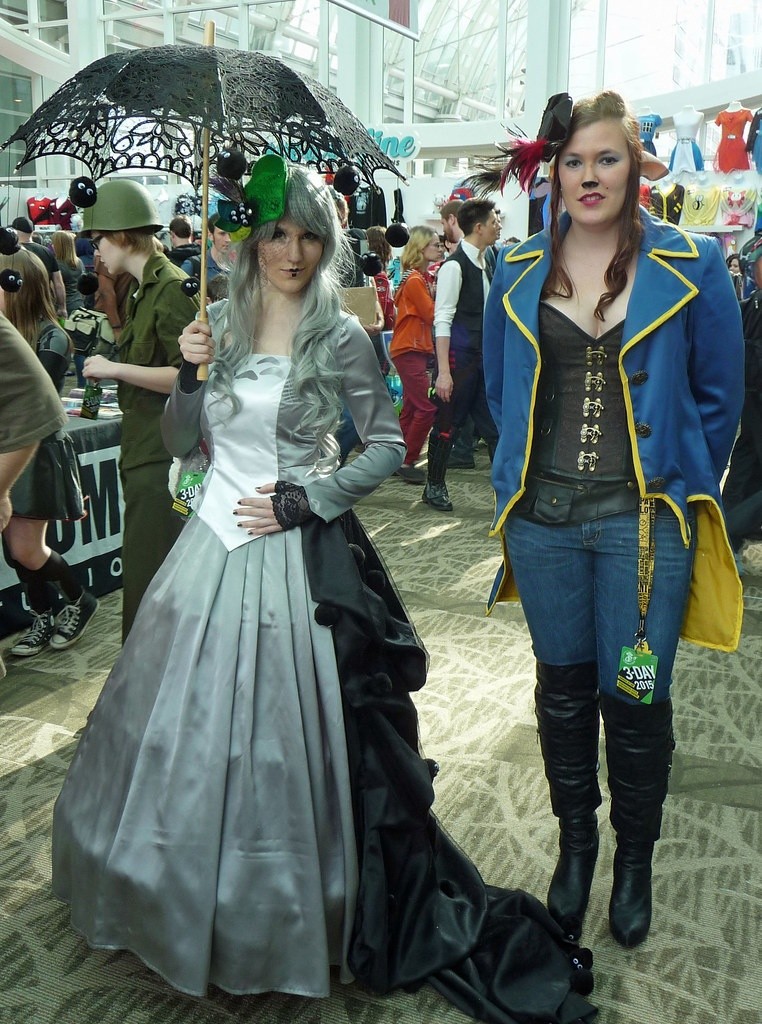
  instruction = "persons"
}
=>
[480,91,745,948]
[712,230,762,578]
[668,105,705,171]
[49,156,596,1023]
[365,197,520,512]
[713,102,753,174]
[633,107,661,157]
[0,180,236,655]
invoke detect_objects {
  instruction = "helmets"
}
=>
[78,179,164,242]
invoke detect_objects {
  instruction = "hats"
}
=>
[10,215,34,233]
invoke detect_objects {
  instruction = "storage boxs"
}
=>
[341,287,378,327]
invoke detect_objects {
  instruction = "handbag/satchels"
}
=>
[65,306,115,357]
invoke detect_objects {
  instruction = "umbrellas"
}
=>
[0,21,408,382]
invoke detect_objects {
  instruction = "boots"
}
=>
[599,686,676,949]
[534,659,603,927]
[421,432,454,511]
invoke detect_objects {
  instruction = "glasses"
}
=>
[88,233,105,249]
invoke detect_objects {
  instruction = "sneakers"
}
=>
[10,608,55,656]
[49,590,101,649]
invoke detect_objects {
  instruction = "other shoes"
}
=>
[448,452,484,469]
[392,465,424,482]
